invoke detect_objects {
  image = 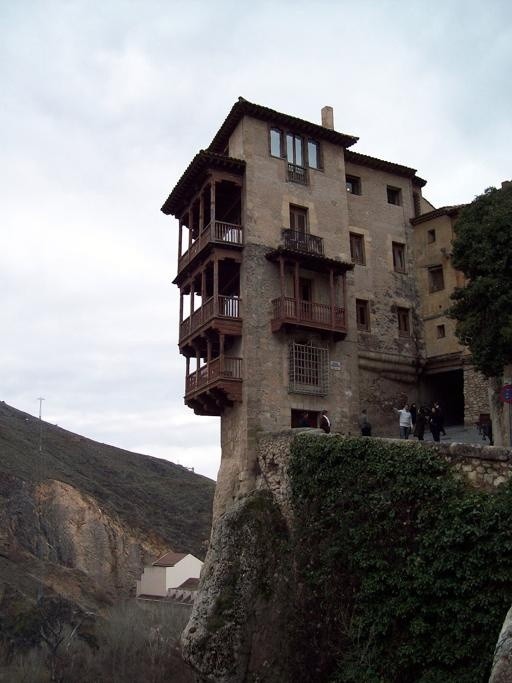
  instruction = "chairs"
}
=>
[473,413,489,434]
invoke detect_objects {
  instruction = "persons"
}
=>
[391,400,448,443]
[360,416,372,437]
[299,410,311,427]
[358,409,372,427]
[320,409,332,433]
